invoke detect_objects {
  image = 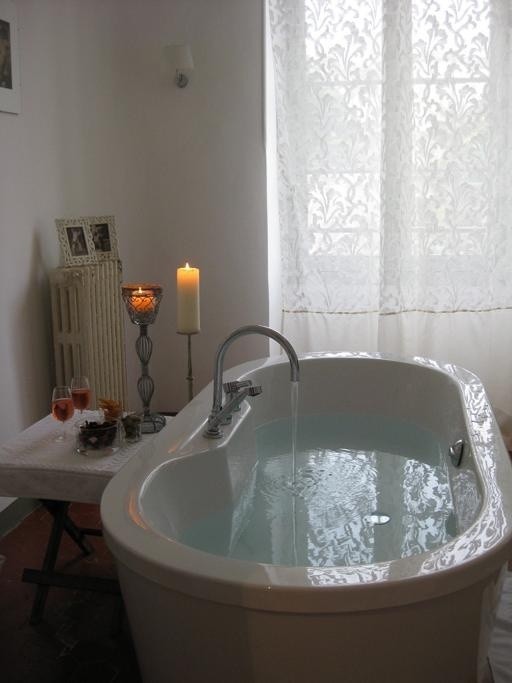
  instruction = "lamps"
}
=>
[173,45,192,87]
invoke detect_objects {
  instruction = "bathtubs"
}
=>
[99,347,512,683]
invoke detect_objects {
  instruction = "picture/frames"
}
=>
[57,219,97,265]
[87,216,116,258]
[0,8,22,115]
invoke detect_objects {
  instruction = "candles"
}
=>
[131,286,156,312]
[176,261,201,330]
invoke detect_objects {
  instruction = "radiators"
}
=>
[54,257,125,408]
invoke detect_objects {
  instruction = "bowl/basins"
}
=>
[74,416,122,459]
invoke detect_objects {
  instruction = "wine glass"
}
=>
[51,374,91,442]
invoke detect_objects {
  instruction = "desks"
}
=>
[1,411,173,621]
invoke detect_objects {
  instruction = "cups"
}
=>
[121,413,142,445]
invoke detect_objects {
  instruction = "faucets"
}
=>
[204,321,301,427]
